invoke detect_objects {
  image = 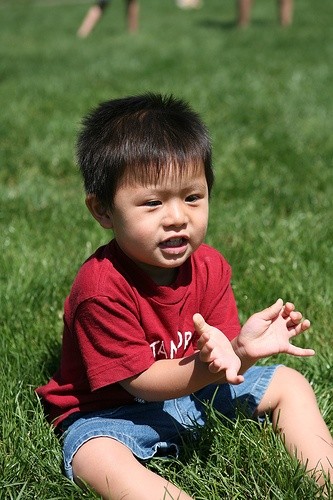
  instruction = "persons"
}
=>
[234,0,294,37]
[35,91,333,500]
[76,0,142,43]
